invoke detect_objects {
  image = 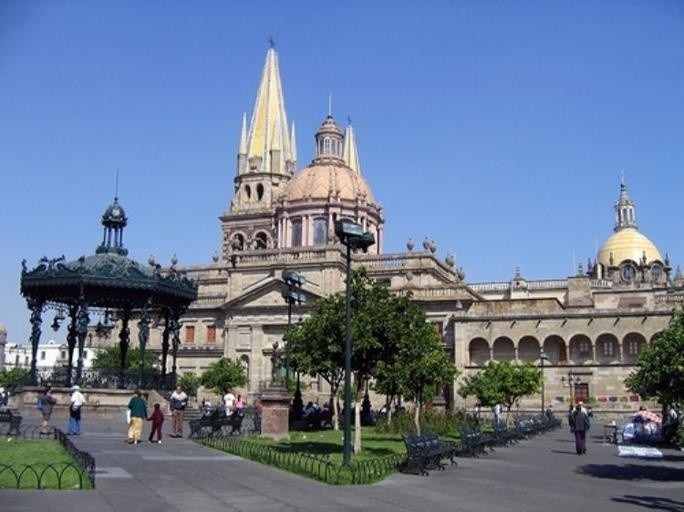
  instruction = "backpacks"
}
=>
[38,396,50,409]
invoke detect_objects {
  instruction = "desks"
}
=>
[603,423,618,444]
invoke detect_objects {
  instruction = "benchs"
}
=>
[293,408,391,428]
[0,407,24,437]
[188,416,243,437]
[399,411,564,475]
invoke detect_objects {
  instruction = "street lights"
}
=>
[281,268,306,391]
[539,352,550,414]
[562,370,582,411]
[334,217,376,463]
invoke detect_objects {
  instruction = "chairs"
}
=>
[612,427,626,442]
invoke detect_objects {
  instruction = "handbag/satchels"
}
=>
[127,410,131,424]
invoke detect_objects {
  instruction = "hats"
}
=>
[70,385,80,390]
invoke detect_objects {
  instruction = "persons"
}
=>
[124,390,147,445]
[197,382,406,431]
[38,385,57,435]
[146,403,165,445]
[65,385,86,436]
[546,399,680,455]
[168,383,189,438]
[491,402,501,425]
